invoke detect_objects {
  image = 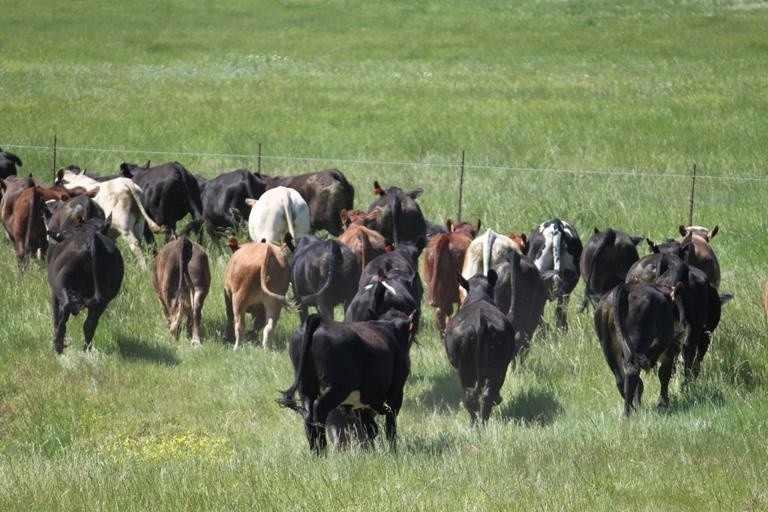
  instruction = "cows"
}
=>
[1,148,735,459]
